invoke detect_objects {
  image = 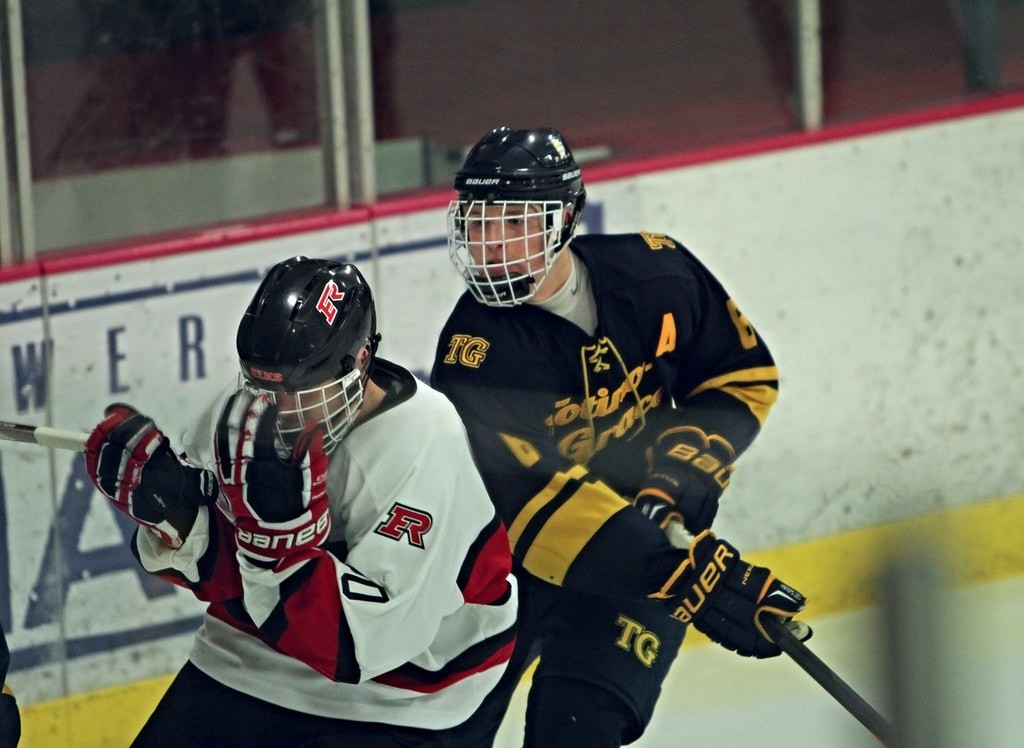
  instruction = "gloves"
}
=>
[213,389,331,574]
[85,402,200,549]
[645,529,813,658]
[633,427,734,536]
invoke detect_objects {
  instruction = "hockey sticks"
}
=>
[0,419,91,454]
[663,519,910,748]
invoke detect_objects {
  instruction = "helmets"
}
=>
[235,256,377,451]
[445,126,586,307]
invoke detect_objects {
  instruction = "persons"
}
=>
[86,256,518,748]
[76,1,399,156]
[430,127,813,748]
